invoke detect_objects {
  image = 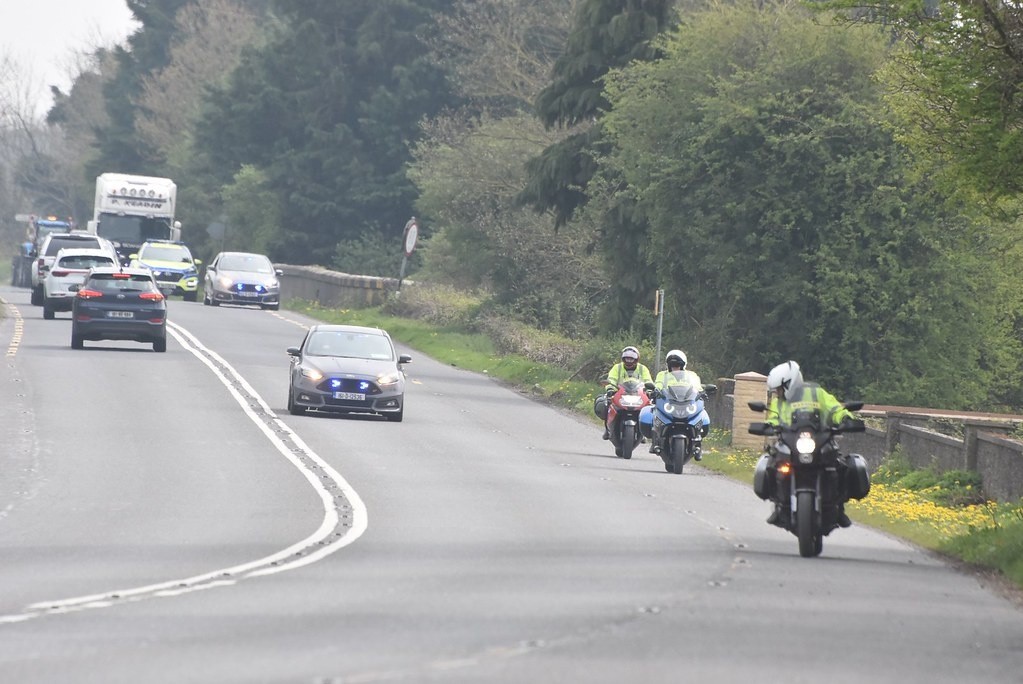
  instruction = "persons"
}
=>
[649,349,709,461]
[602,346,654,443]
[767,360,861,529]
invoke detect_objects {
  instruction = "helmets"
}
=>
[621,346,640,370]
[766,360,803,402]
[665,350,687,371]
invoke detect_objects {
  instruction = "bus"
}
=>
[86,172,177,270]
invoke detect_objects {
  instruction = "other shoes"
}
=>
[828,505,852,527]
[767,502,791,523]
[641,436,647,443]
[695,443,702,461]
[603,431,609,440]
[649,443,657,453]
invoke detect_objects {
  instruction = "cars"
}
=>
[286,324,412,421]
[203,250,284,310]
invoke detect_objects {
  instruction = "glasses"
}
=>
[668,361,685,368]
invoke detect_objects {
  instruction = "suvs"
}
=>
[40,247,123,321]
[30,230,124,306]
[69,265,172,352]
[128,238,203,303]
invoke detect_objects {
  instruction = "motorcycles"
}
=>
[639,381,717,475]
[593,377,656,460]
[746,400,871,557]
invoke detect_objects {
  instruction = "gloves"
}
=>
[607,388,615,397]
[651,390,657,398]
[842,414,852,423]
[699,391,709,402]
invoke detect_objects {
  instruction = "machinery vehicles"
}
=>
[10,214,73,287]
